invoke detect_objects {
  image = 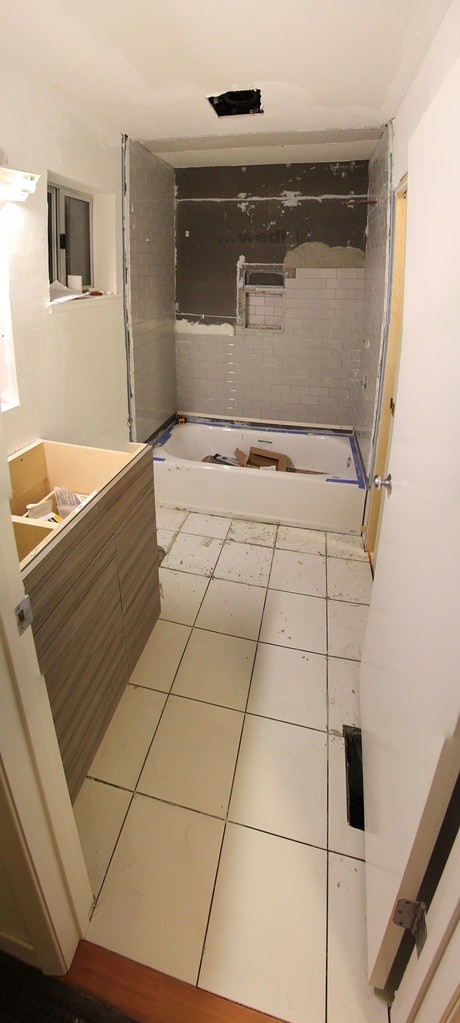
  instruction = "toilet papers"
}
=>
[68,275,82,292]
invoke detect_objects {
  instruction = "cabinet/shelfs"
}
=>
[8,438,161,806]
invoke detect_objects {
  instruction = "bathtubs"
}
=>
[151,421,367,535]
